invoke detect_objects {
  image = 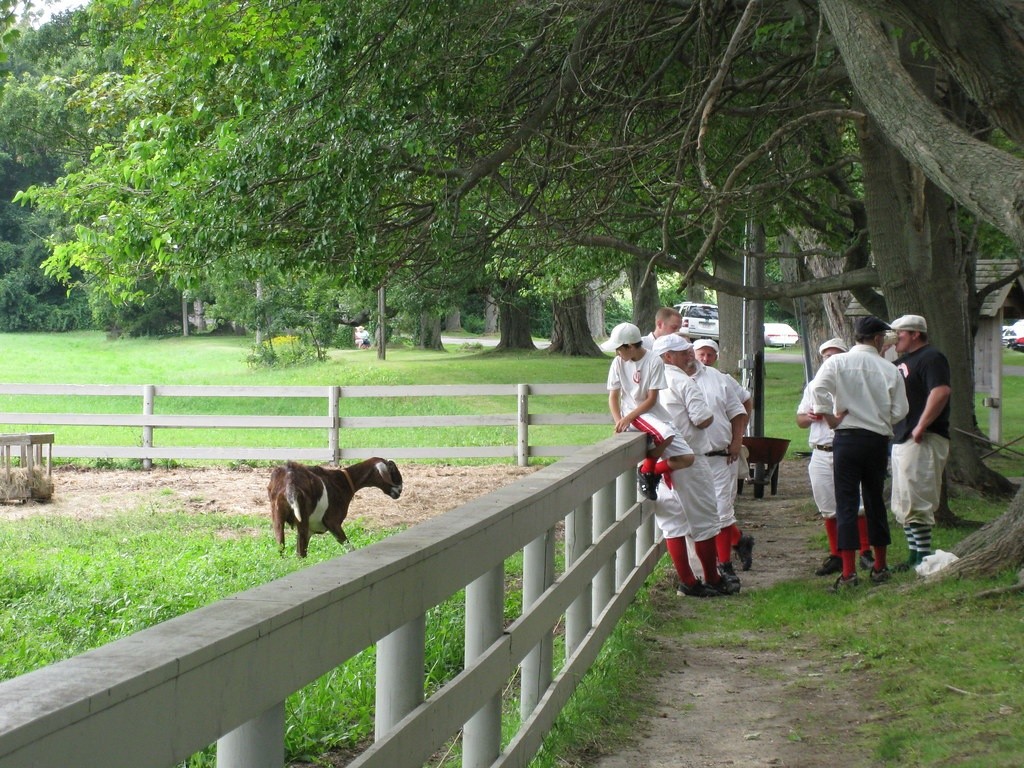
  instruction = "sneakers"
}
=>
[815,555,842,577]
[860,550,875,572]
[719,562,741,594]
[869,567,891,585]
[713,576,729,595]
[678,576,718,597]
[732,530,755,571]
[827,573,862,594]
[638,464,661,500]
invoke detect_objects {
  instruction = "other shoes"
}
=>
[890,563,910,573]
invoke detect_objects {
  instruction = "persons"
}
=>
[600,322,695,502]
[641,307,682,350]
[811,315,910,597]
[879,314,951,574]
[795,338,874,575]
[355,326,369,348]
[647,332,755,596]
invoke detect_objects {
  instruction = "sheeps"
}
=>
[269,456,402,558]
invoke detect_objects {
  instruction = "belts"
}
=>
[834,429,877,436]
[816,445,834,452]
[705,449,729,457]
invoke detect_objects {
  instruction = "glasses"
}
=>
[672,350,688,354]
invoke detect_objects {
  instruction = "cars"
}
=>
[1002,319,1024,352]
[764,322,799,347]
[673,301,719,343]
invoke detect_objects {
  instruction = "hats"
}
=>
[693,339,719,352]
[819,338,848,355]
[653,334,693,356]
[856,316,892,334]
[891,314,927,332]
[601,323,642,350]
